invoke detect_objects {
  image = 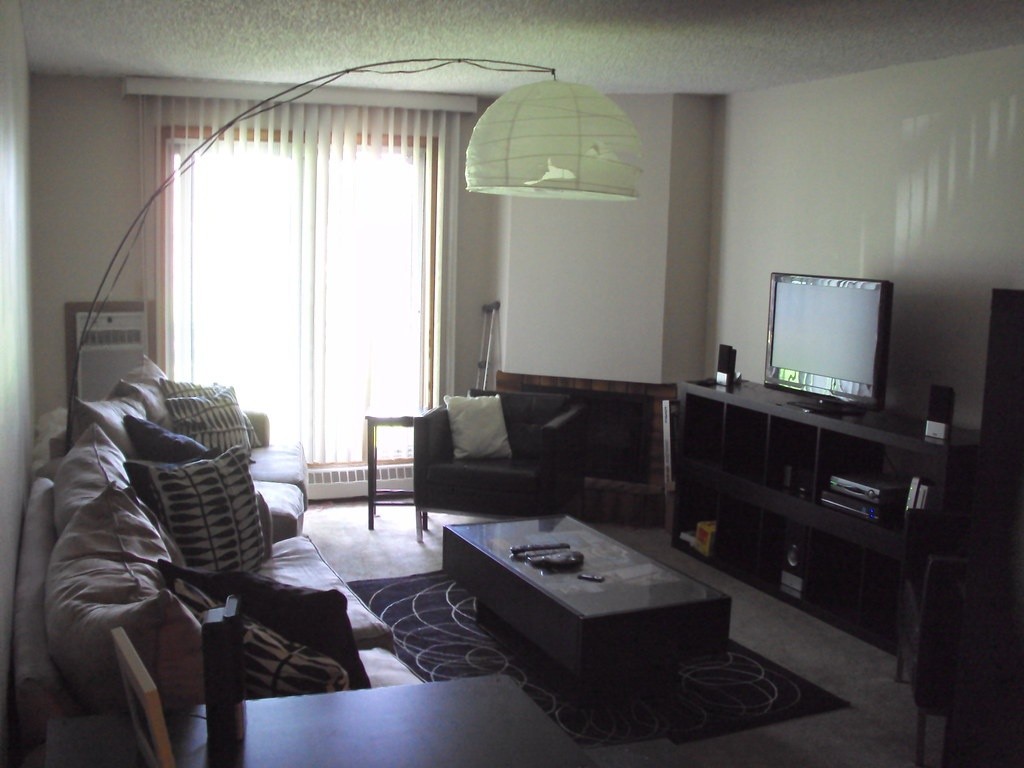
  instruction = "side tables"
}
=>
[41,672,601,768]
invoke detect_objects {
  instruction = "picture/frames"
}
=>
[107,623,178,768]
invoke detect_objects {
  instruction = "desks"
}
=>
[364,413,431,532]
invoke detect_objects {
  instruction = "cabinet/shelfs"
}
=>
[669,376,984,659]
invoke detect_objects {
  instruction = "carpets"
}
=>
[347,569,852,748]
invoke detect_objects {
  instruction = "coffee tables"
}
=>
[440,512,735,686]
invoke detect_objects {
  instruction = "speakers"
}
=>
[926,386,954,440]
[716,344,737,387]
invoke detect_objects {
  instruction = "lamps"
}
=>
[61,57,643,453]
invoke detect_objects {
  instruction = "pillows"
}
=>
[52,421,131,537]
[443,394,513,460]
[122,443,264,575]
[172,578,351,698]
[156,556,375,689]
[44,478,207,708]
[120,351,175,432]
[124,413,208,465]
[69,393,148,455]
[158,376,263,448]
[163,386,252,463]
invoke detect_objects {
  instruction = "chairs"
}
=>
[413,389,590,530]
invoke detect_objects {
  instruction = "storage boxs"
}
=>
[780,570,803,592]
[693,520,717,558]
[679,531,696,544]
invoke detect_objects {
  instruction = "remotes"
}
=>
[510,542,583,567]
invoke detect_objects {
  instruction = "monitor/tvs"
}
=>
[764,272,893,414]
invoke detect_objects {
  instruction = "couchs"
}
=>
[0,400,425,768]
